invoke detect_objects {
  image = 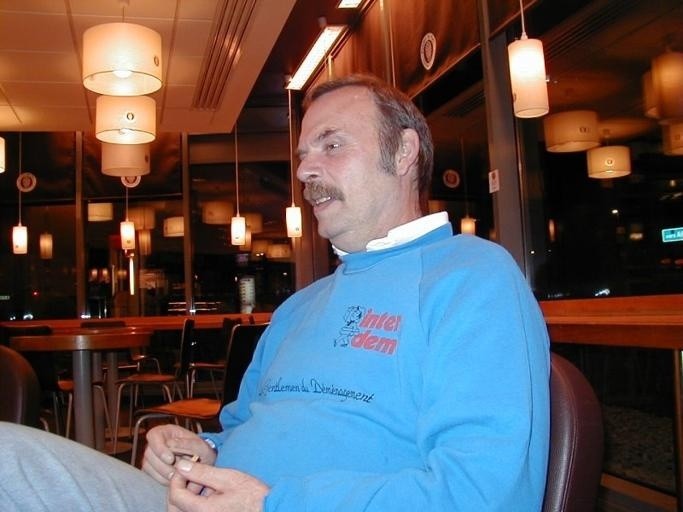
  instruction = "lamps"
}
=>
[197,196,297,264]
[11,128,28,255]
[456,132,479,237]
[641,40,683,157]
[100,141,151,177]
[94,93,159,145]
[586,129,633,181]
[541,106,600,154]
[230,119,246,247]
[79,0,165,98]
[118,175,138,250]
[85,201,185,293]
[281,15,352,93]
[505,2,553,120]
[282,74,304,239]
[39,206,56,260]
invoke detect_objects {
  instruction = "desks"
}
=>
[535,291,682,361]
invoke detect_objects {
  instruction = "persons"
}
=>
[1,75,549,510]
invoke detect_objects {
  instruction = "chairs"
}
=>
[544,351,602,510]
[5,312,275,463]
